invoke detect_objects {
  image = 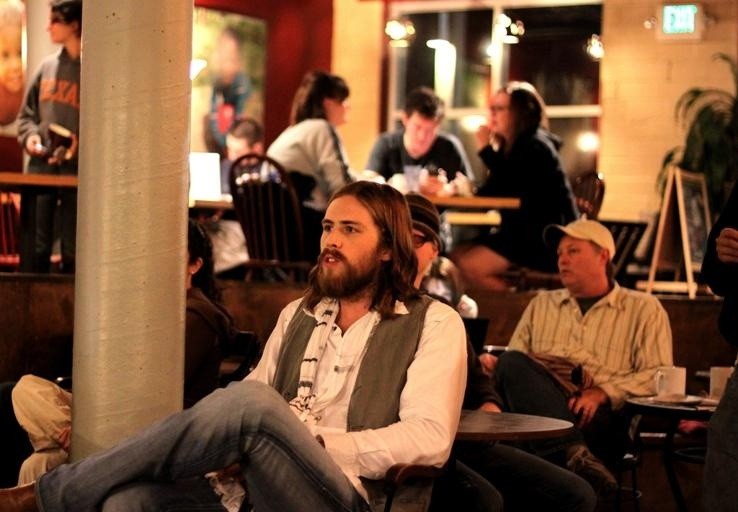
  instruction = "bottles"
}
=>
[403,163,449,199]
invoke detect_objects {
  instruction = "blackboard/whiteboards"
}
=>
[674,166,713,273]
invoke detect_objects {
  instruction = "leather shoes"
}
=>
[0,481,38,512]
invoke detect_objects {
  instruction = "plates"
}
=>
[652,395,701,406]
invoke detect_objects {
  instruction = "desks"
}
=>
[426,194,521,253]
[625,392,719,512]
[456,409,573,443]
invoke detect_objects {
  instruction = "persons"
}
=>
[14,1,82,273]
[261,67,352,214]
[476,80,579,266]
[220,118,264,193]
[700,179,737,512]
[364,87,475,184]
[497,219,673,494]
[0,181,469,511]
[204,27,263,159]
[9,217,238,486]
[382,193,504,421]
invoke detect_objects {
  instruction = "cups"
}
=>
[30,118,59,154]
[652,366,686,400]
[707,365,734,400]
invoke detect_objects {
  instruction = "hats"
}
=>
[405,192,446,252]
[543,219,616,262]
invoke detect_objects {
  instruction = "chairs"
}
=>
[229,152,314,283]
[361,445,455,511]
[576,174,604,218]
[600,219,648,279]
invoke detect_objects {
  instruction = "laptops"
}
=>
[189,150,233,199]
